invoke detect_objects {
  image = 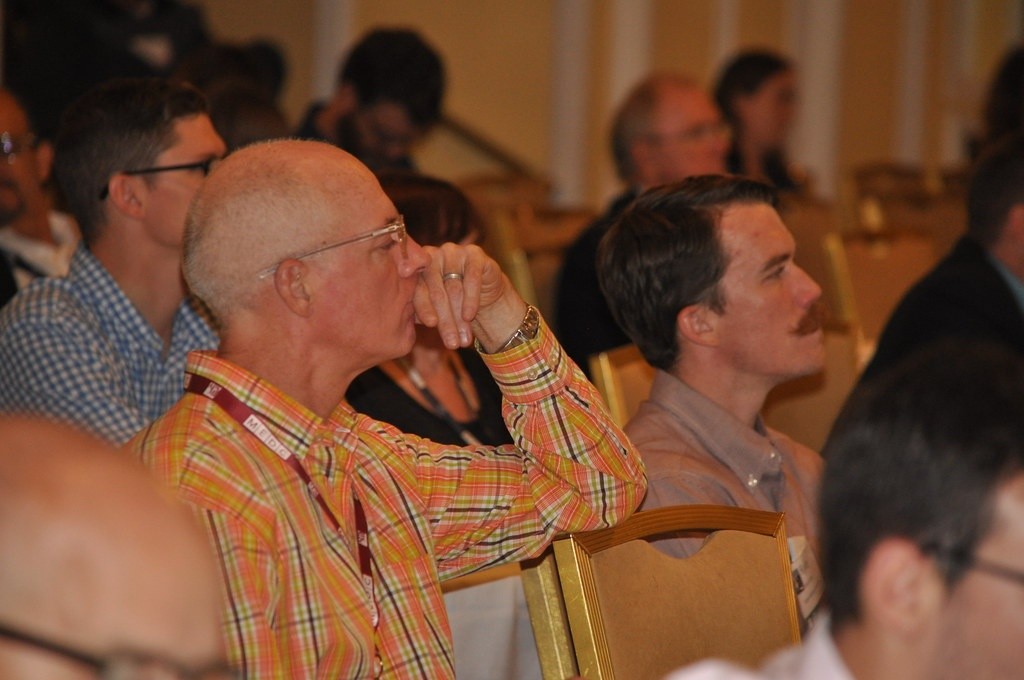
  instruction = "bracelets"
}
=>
[496,301,539,354]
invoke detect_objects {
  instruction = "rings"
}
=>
[442,273,465,284]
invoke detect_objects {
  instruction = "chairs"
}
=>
[448,162,971,680]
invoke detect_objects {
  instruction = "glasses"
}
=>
[0,623,236,680]
[644,118,729,152]
[99,155,219,201]
[257,212,410,281]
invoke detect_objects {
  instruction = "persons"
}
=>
[0,0,1024,680]
